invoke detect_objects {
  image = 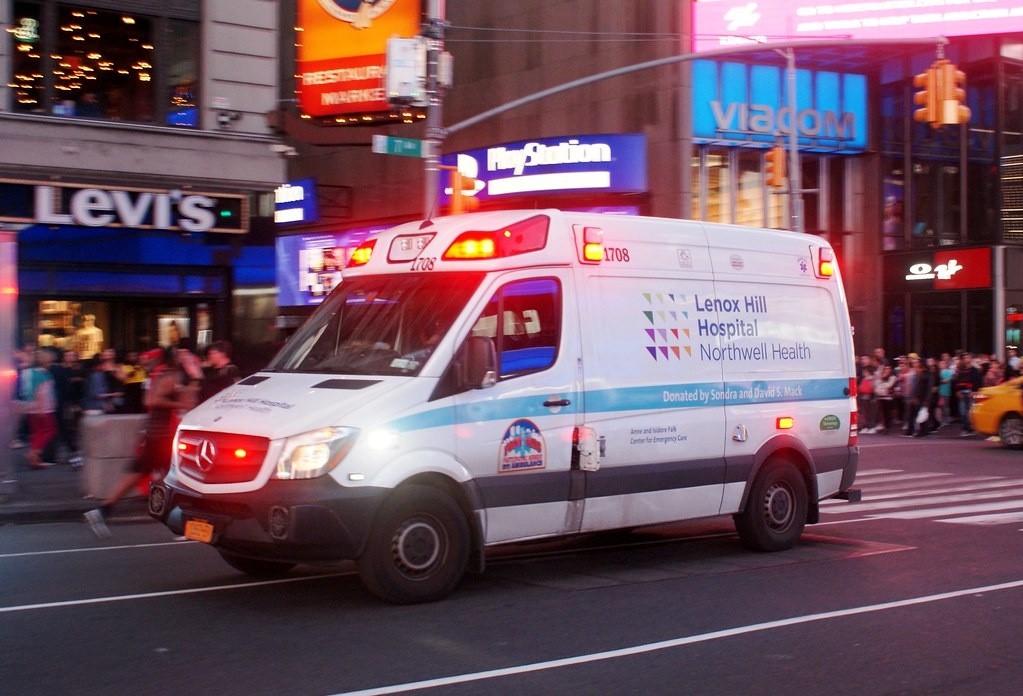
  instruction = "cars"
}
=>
[970,375,1023,451]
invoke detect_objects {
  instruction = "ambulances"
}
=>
[148,208,860,604]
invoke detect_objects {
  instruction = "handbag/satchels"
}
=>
[915,406,929,423]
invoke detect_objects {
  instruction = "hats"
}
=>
[907,353,919,359]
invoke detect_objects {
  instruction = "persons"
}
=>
[74,91,104,118]
[10,337,242,541]
[856,348,1023,442]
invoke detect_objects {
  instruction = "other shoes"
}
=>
[914,432,928,439]
[83,509,112,540]
[959,430,976,437]
[9,439,23,449]
[868,428,879,434]
[901,424,909,430]
[858,427,868,434]
[891,418,903,424]
[24,451,46,468]
[900,432,912,437]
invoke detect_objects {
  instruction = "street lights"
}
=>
[709,32,804,233]
